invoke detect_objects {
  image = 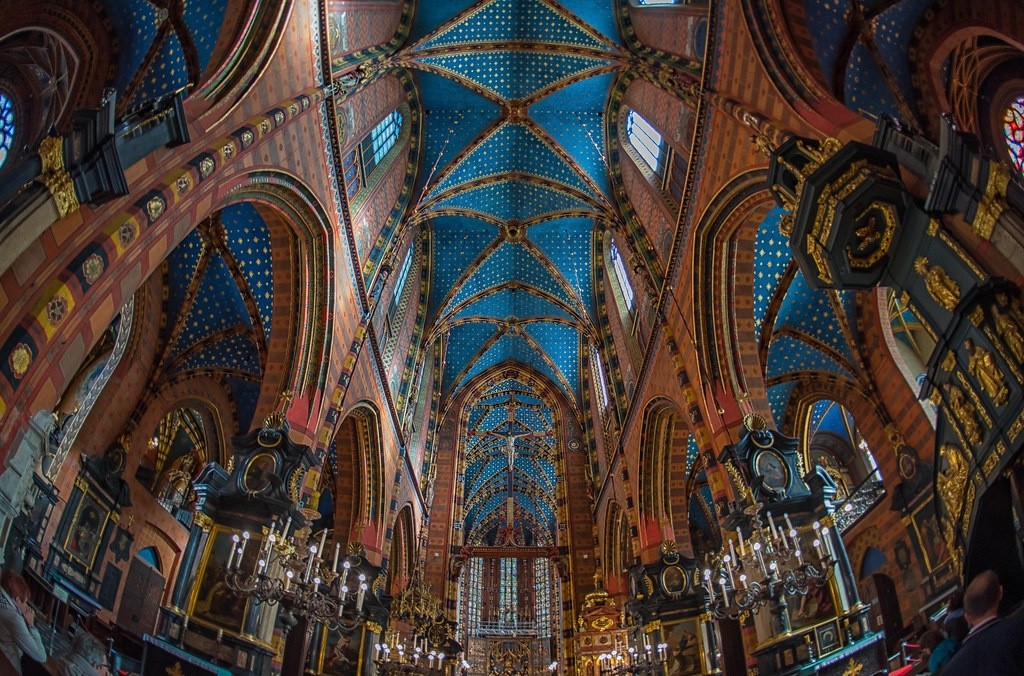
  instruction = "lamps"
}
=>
[702,510,841,621]
[223,517,368,633]
[598,634,668,676]
[373,631,470,676]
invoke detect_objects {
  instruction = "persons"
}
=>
[51,631,111,676]
[0,569,46,676]
[252,461,268,482]
[920,630,961,673]
[940,570,1024,676]
[158,454,194,502]
